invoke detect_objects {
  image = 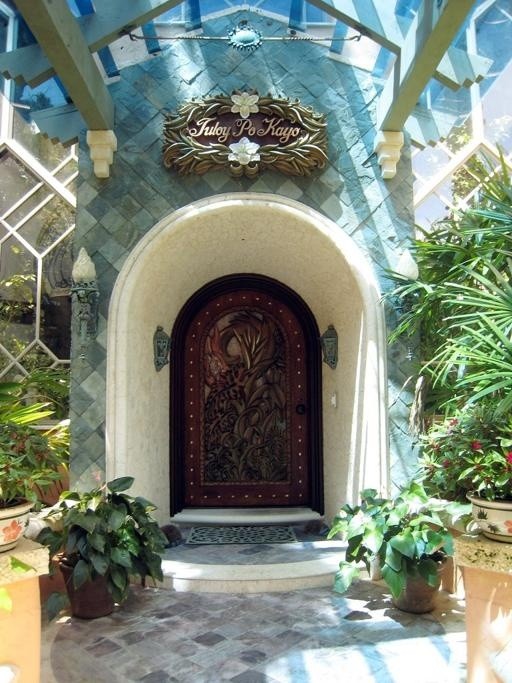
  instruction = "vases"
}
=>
[464,488,512,543]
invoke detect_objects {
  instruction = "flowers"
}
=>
[415,378,512,500]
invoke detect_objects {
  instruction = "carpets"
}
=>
[183,524,300,545]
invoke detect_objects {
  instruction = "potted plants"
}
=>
[321,476,461,615]
[36,475,173,619]
[1,380,75,557]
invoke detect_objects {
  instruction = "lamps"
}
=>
[66,244,102,366]
[317,322,338,370]
[152,323,174,373]
[393,247,422,365]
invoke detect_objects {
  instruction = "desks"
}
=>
[1,535,53,683]
[449,526,512,683]
